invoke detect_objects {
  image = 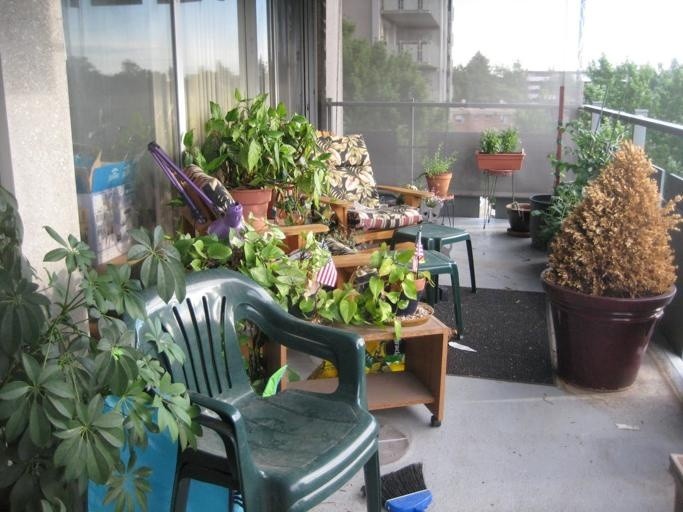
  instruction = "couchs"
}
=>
[301,130,435,251]
[170,162,331,263]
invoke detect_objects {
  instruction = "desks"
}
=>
[267,296,452,426]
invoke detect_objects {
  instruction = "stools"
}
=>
[433,195,457,226]
[391,249,465,340]
[394,220,478,302]
[479,169,517,230]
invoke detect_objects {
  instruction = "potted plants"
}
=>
[345,242,436,326]
[505,202,534,238]
[415,142,458,197]
[540,139,683,394]
[182,88,332,231]
[473,126,526,171]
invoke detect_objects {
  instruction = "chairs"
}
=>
[119,269,384,512]
